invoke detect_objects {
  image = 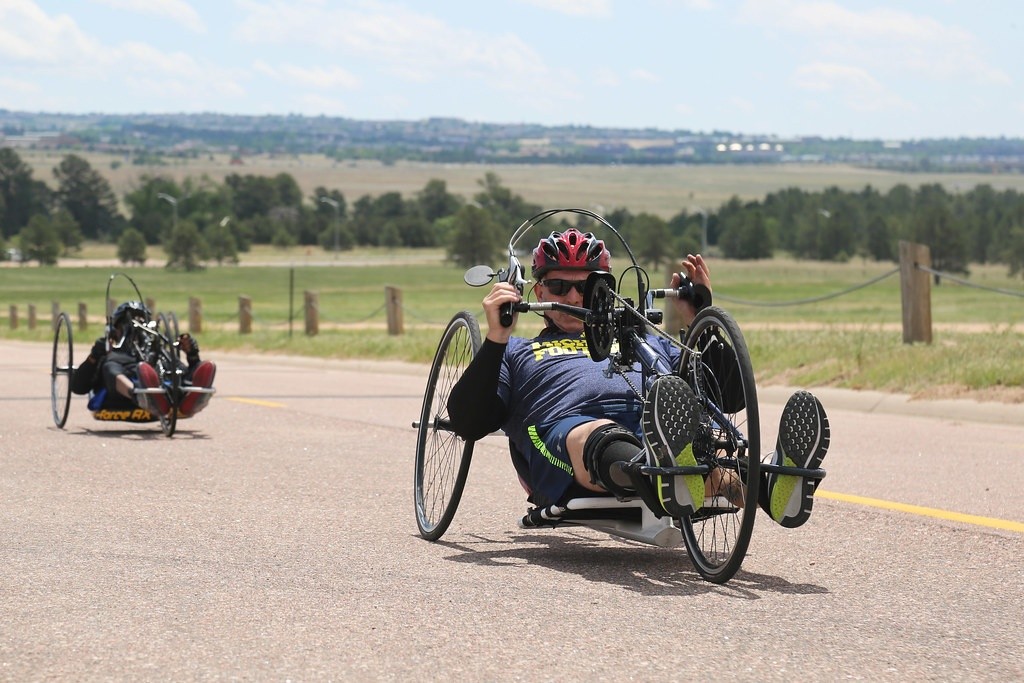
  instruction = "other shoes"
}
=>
[137,362,171,417]
[640,376,705,519]
[757,390,831,527]
[180,361,216,416]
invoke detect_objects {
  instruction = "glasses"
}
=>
[540,279,587,298]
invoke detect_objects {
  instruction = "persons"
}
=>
[71,299,216,417]
[446,226,831,528]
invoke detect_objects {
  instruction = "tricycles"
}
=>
[50,271,218,437]
[409,207,828,585]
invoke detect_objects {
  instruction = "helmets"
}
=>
[531,228,612,282]
[113,301,151,329]
